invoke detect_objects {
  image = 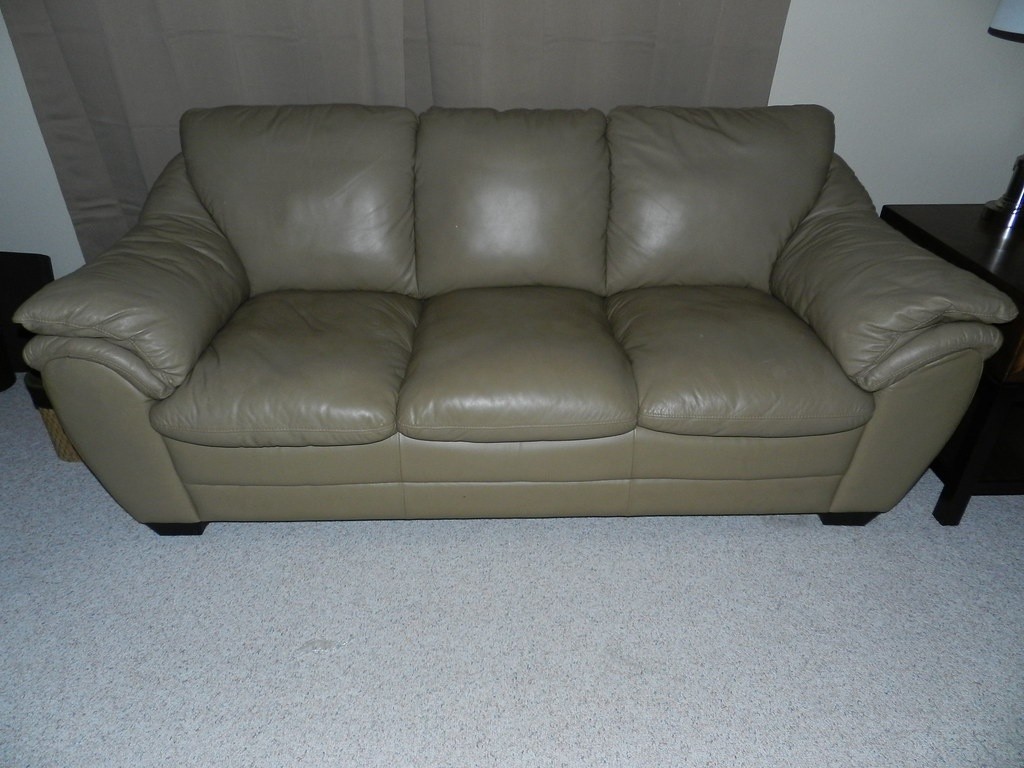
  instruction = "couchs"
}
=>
[13,101,1015,536]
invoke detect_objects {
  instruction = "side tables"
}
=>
[876,200,1024,528]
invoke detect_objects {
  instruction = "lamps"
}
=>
[987,0,1024,216]
